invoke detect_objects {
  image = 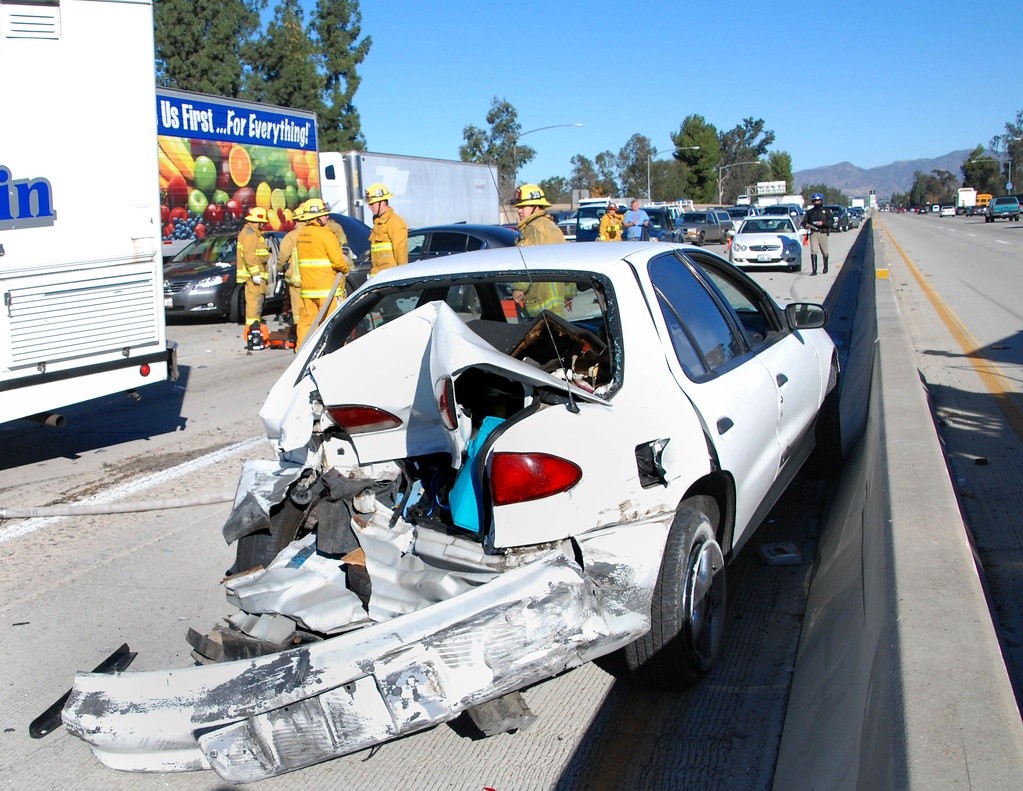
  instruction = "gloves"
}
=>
[253,275,262,284]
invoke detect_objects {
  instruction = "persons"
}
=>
[512,184,576,320]
[622,201,650,241]
[237,207,270,342]
[802,193,834,276]
[598,203,624,241]
[276,199,354,355]
[368,183,408,280]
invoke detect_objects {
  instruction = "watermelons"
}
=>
[242,144,289,190]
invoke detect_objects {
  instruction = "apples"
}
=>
[187,139,253,216]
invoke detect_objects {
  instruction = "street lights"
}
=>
[718,161,761,204]
[972,160,1011,195]
[647,146,699,200]
[514,123,582,189]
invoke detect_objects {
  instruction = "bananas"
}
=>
[157,135,196,194]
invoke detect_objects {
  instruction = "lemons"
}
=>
[256,182,272,210]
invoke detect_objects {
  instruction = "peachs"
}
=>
[158,173,205,237]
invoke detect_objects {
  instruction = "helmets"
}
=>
[292,207,304,220]
[511,184,552,207]
[247,207,267,222]
[365,184,394,205]
[811,193,824,203]
[607,203,617,210]
[300,199,329,222]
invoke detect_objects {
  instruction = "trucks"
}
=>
[1,0,178,443]
[157,85,320,264]
[319,149,499,253]
[753,195,804,212]
[954,187,977,206]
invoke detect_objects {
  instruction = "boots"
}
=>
[811,254,817,276]
[823,256,828,273]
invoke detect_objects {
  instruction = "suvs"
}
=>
[550,197,808,244]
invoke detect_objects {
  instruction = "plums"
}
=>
[205,200,242,223]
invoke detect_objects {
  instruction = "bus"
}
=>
[976,193,992,206]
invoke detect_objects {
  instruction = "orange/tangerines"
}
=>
[229,144,320,230]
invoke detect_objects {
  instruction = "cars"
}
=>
[730,214,812,272]
[29,242,840,784]
[824,205,865,232]
[164,214,518,322]
[736,194,751,206]
[910,194,1023,222]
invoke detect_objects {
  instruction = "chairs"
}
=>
[747,221,759,230]
[776,221,787,230]
